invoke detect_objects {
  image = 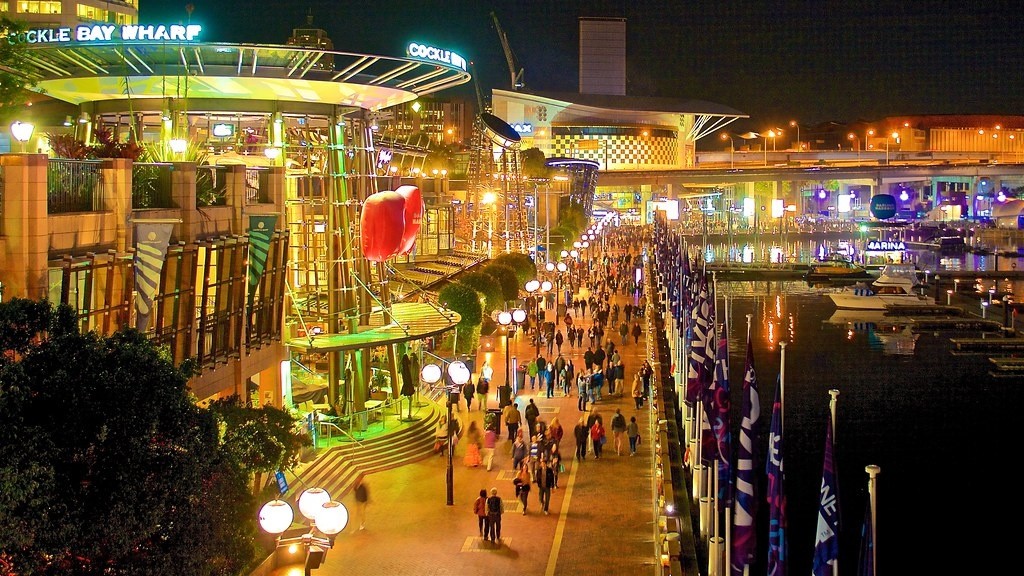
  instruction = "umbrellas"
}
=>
[408,353,428,407]
[399,354,421,422]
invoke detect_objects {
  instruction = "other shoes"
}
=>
[578,409,588,412]
[491,540,495,544]
[608,392,623,397]
[544,511,549,515]
[477,442,482,449]
[540,503,543,509]
[497,537,499,539]
[555,486,559,488]
[531,384,572,398]
[522,509,526,515]
[614,449,618,452]
[484,537,488,540]
[600,433,606,443]
[618,453,624,456]
[586,398,602,404]
[577,451,603,462]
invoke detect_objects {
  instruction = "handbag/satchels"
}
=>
[615,414,623,428]
[630,452,635,457]
[559,464,565,473]
[581,393,587,397]
[434,430,458,452]
[636,432,641,445]
[526,364,530,375]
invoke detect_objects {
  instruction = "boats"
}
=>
[822,306,920,358]
[802,255,936,309]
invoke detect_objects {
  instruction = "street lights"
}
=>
[701,205,797,247]
[525,280,553,357]
[422,360,471,506]
[259,487,348,576]
[545,261,567,325]
[886,133,899,165]
[722,133,733,168]
[490,308,526,386]
[790,120,800,153]
[848,133,861,168]
[560,250,579,294]
[865,130,874,150]
[573,219,608,286]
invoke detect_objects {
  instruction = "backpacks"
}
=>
[548,333,553,341]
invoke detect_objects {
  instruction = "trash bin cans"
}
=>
[573,283,579,295]
[543,322,554,339]
[581,269,587,279]
[558,304,567,317]
[484,409,503,435]
[593,257,597,264]
[496,385,512,409]
[517,368,526,390]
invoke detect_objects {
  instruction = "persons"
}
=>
[436,223,653,485]
[517,463,532,516]
[485,487,504,543]
[474,490,489,541]
[351,478,367,535]
[537,460,555,515]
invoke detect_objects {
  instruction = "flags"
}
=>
[811,397,838,576]
[857,480,874,576]
[765,376,789,576]
[731,335,761,576]
[653,213,734,514]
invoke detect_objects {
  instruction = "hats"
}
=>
[490,488,497,494]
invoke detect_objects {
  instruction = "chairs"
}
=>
[297,395,328,413]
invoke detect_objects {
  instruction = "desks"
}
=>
[314,404,328,409]
[364,400,383,422]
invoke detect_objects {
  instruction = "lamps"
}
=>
[401,324,410,331]
[391,166,447,178]
[442,303,448,310]
